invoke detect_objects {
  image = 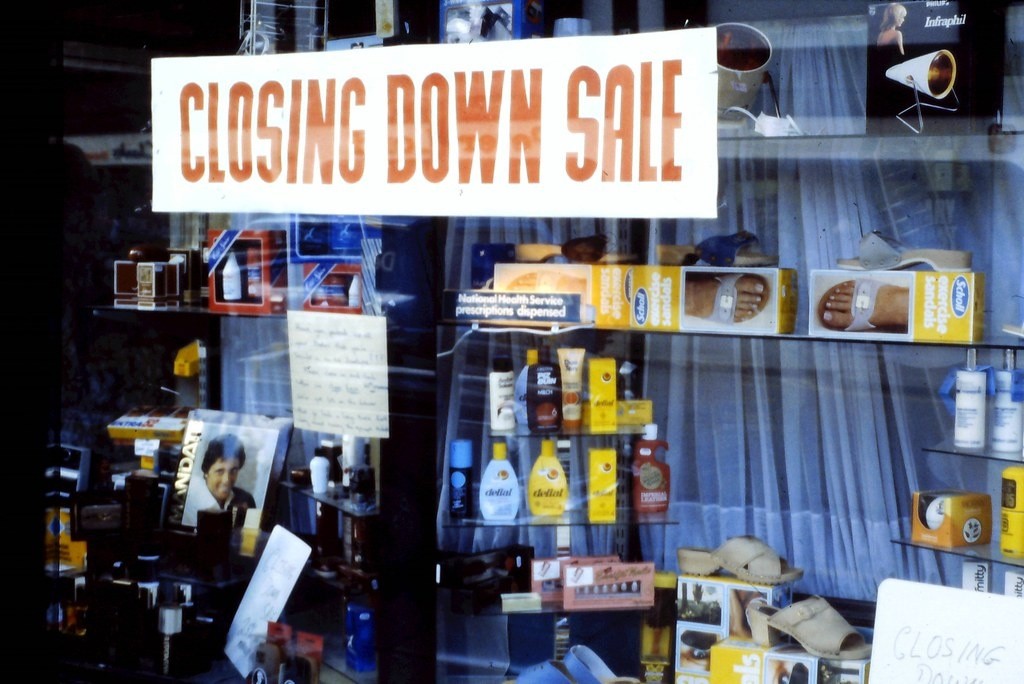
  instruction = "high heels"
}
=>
[677,536,805,584]
[747,593,872,660]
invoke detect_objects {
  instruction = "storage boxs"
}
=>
[911,489,993,548]
[112,245,208,304]
[530,556,655,609]
[805,270,983,347]
[676,573,870,684]
[492,263,799,336]
[206,228,287,314]
[582,358,653,433]
[586,447,616,523]
[106,405,196,445]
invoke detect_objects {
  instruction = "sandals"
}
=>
[815,281,910,331]
[685,273,769,324]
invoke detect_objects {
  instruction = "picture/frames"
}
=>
[161,407,292,561]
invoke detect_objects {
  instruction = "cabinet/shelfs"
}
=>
[0,0,1024,684]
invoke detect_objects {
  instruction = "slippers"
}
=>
[502,644,638,684]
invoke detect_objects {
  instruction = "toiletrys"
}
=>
[996,461,1024,561]
[300,268,366,309]
[471,347,651,430]
[220,242,265,299]
[938,341,1024,457]
[445,422,672,522]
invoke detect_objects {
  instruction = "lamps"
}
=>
[714,20,783,131]
